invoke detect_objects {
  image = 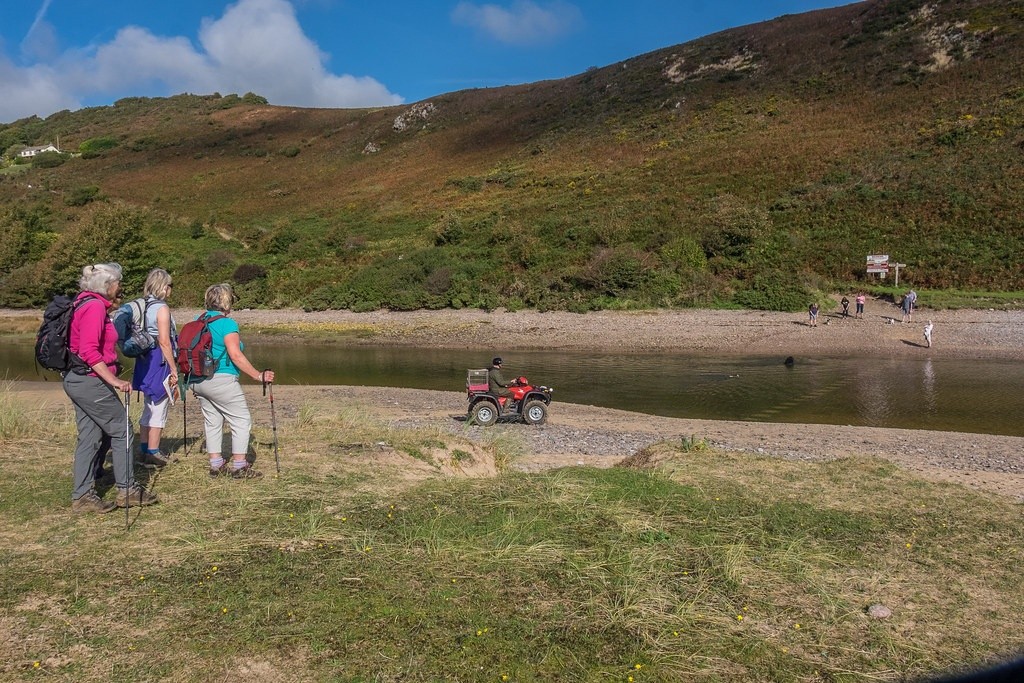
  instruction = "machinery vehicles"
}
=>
[466,369,552,427]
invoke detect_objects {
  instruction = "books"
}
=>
[163,373,180,406]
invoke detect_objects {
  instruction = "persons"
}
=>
[856,291,865,319]
[488,357,517,413]
[841,297,851,319]
[809,303,819,327]
[900,289,917,323]
[63,262,159,513]
[923,320,933,347]
[132,268,179,468]
[190,283,275,481]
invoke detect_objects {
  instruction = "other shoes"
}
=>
[231,466,262,478]
[503,406,513,413]
[102,469,112,476]
[209,464,231,479]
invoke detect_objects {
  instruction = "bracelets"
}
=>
[258,374,261,380]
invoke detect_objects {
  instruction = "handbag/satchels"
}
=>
[97,361,126,377]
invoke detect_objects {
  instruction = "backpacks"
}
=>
[108,298,171,358]
[177,312,227,376]
[34,295,105,377]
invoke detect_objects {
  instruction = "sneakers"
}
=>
[144,452,179,469]
[73,491,116,513]
[116,487,157,507]
[135,451,145,466]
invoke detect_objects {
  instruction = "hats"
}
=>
[493,357,503,365]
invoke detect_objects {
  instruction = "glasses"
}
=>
[116,295,123,299]
[167,283,173,288]
